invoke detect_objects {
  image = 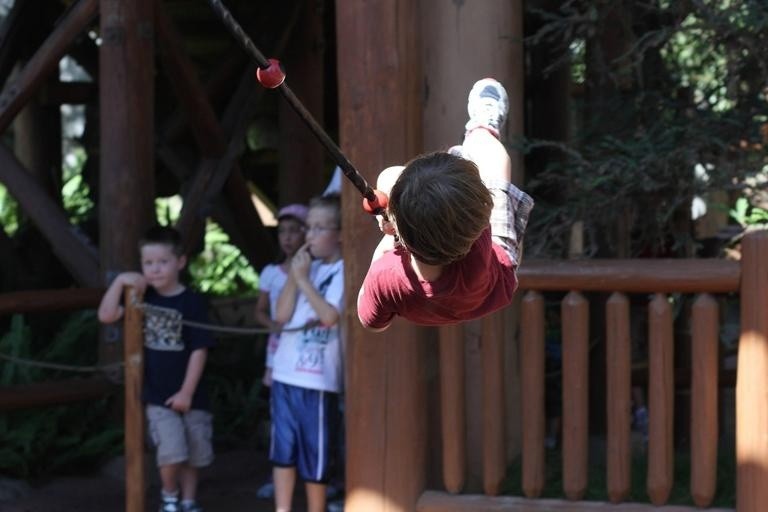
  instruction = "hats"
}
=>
[464,78,509,140]
[277,205,311,224]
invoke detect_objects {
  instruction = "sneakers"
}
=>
[160,496,201,512]
[256,475,275,499]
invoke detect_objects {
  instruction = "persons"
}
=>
[255,203,337,497]
[267,191,345,510]
[97,228,217,511]
[356,78,535,333]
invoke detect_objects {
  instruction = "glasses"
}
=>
[303,225,341,235]
